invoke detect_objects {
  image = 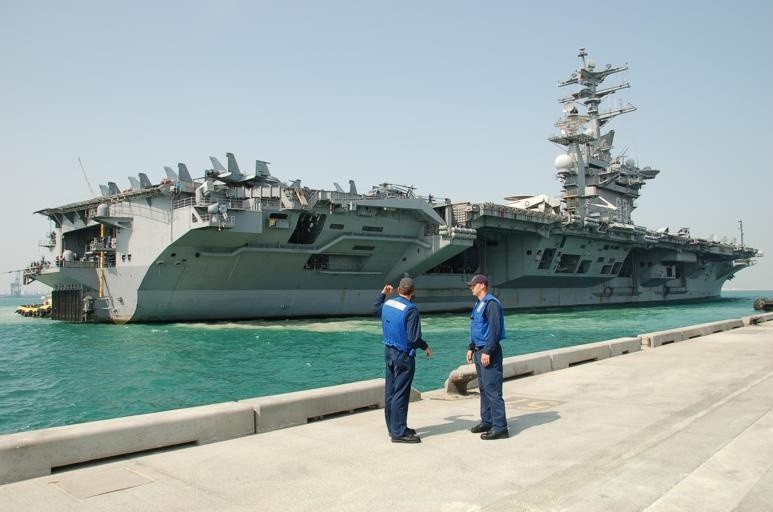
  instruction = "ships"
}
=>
[23,45,764,326]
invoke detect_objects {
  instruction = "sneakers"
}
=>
[401,429,416,436]
[471,422,490,433]
[392,435,420,443]
[481,430,508,439]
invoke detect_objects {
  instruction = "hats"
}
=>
[465,274,488,285]
[400,278,414,292]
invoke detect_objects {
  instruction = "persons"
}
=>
[374,278,432,443]
[464,275,509,440]
